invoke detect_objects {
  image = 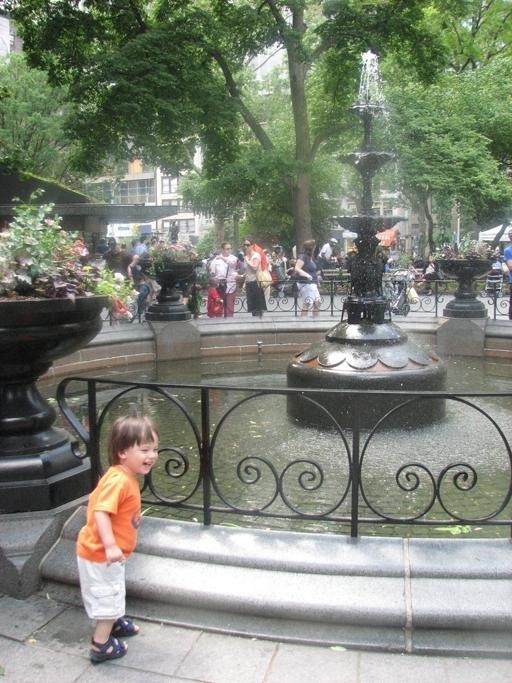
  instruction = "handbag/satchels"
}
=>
[256,270,273,287]
[217,279,227,293]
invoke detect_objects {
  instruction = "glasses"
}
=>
[244,243,250,247]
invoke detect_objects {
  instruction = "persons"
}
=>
[503,232,512,320]
[75,414,161,664]
[74,231,338,323]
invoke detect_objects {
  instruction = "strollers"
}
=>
[385,280,411,316]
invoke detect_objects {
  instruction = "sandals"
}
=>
[111,618,138,636]
[90,636,127,662]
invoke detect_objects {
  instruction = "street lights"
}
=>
[399,237,406,252]
[439,225,446,247]
